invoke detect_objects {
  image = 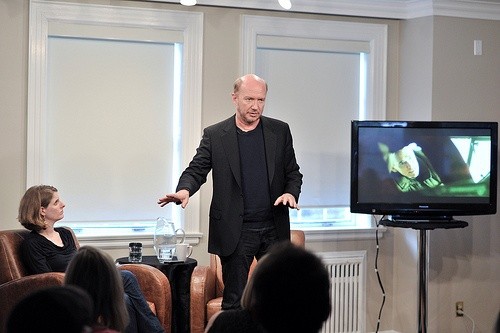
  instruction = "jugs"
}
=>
[154,217,185,263]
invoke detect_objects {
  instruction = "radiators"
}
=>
[306,249,367,333]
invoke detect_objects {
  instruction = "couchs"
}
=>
[0,225,173,333]
[190,229,305,333]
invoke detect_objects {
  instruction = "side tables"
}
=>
[116,255,197,333]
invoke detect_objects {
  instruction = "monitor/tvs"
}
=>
[350,119,498,222]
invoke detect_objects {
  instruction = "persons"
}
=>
[248,247,332,333]
[63,244,130,333]
[204,252,270,333]
[16,184,166,333]
[386,139,449,194]
[5,284,95,333]
[156,75,304,311]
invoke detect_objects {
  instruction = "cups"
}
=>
[175,244,193,262]
[128,242,143,262]
